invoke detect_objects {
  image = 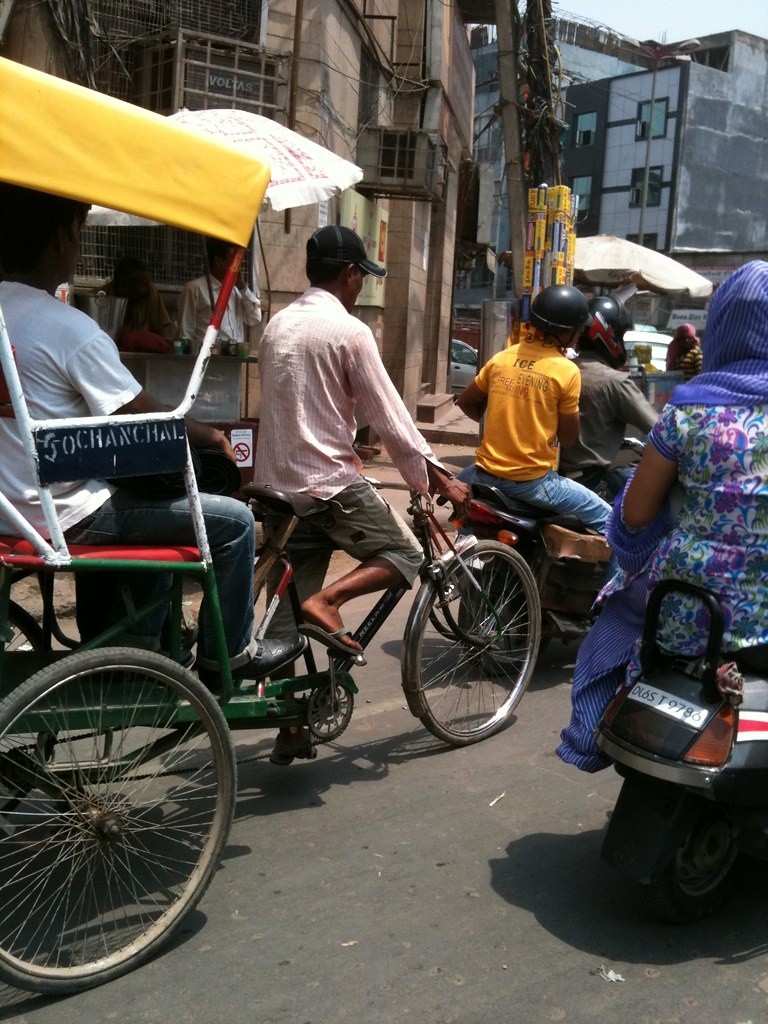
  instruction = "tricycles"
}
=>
[0,58,542,1000]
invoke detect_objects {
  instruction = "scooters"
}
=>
[599,578,767,916]
[448,434,655,680]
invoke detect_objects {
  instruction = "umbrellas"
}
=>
[84,108,365,313]
[574,233,714,299]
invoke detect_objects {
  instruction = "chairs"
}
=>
[0,532,205,579]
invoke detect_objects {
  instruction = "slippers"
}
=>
[269,744,318,766]
[297,624,364,657]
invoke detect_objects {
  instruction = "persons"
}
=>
[621,260,768,681]
[251,225,469,766]
[455,284,612,534]
[0,180,309,691]
[176,239,262,340]
[666,323,703,381]
[558,294,658,505]
[95,255,171,336]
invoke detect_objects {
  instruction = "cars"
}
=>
[623,332,674,373]
[450,338,478,399]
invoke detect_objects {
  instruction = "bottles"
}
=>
[628,355,639,372]
[228,339,237,355]
[173,337,183,354]
[238,343,249,358]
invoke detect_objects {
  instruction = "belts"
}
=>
[558,464,598,480]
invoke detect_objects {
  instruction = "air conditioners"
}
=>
[356,127,435,197]
[136,41,279,122]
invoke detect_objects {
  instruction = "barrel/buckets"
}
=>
[72,291,129,343]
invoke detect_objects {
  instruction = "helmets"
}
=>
[531,284,594,336]
[579,296,633,368]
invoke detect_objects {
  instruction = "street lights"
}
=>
[633,39,704,249]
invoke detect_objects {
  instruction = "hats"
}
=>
[306,225,387,278]
[676,323,700,344]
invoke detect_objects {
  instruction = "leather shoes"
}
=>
[81,650,196,684]
[198,632,308,691]
